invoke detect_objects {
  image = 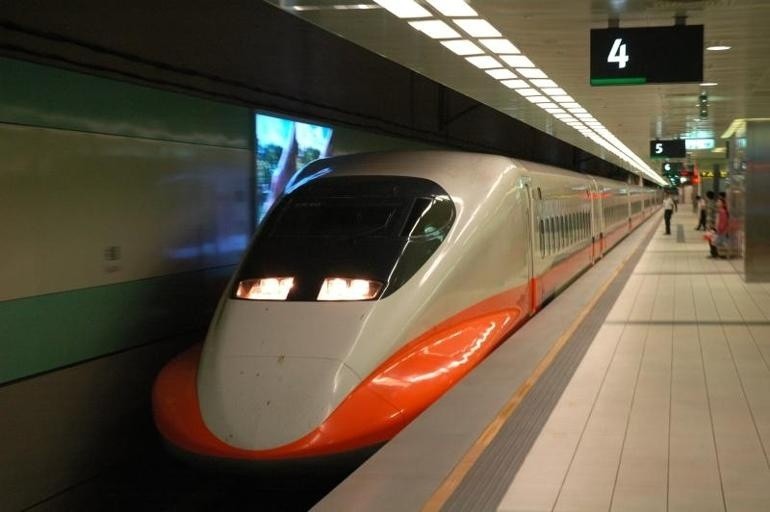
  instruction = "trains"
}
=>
[150,149,668,478]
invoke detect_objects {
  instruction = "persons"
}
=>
[672,191,678,212]
[695,191,728,258]
[663,191,675,234]
